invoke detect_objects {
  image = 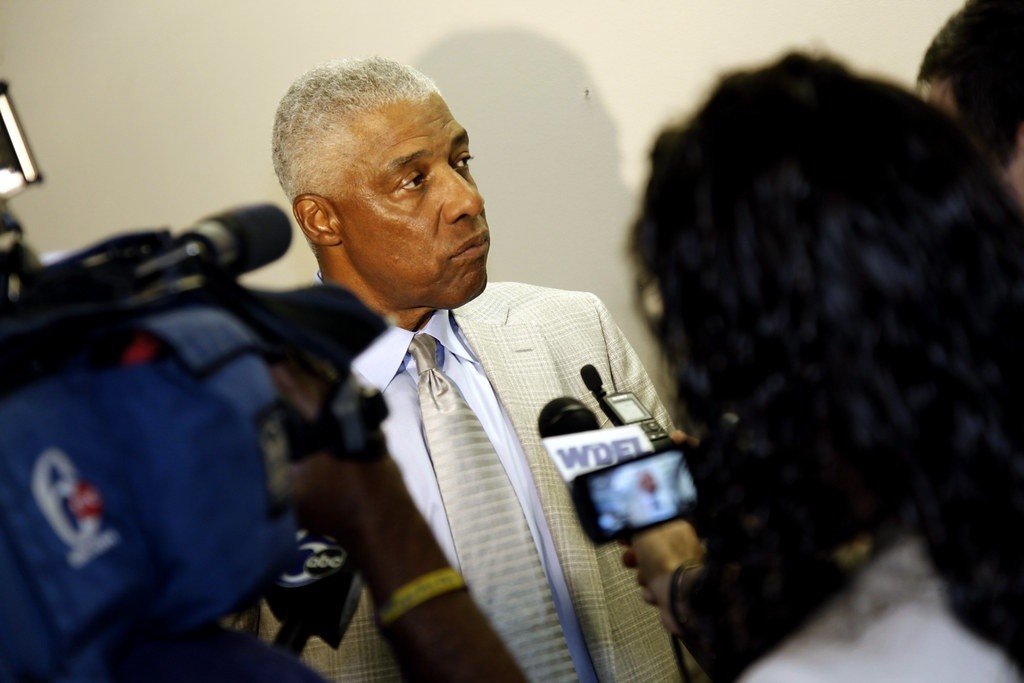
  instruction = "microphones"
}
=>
[261,508,364,659]
[538,395,656,483]
[128,204,292,297]
[579,364,606,401]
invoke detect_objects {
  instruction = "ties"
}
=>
[409,335,583,681]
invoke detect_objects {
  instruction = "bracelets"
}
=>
[377,570,468,631]
[670,561,702,635]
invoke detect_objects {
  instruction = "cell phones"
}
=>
[571,441,705,545]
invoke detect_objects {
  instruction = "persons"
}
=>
[0,308,525,683]
[223,54,705,683]
[618,1,1024,682]
[626,470,677,526]
